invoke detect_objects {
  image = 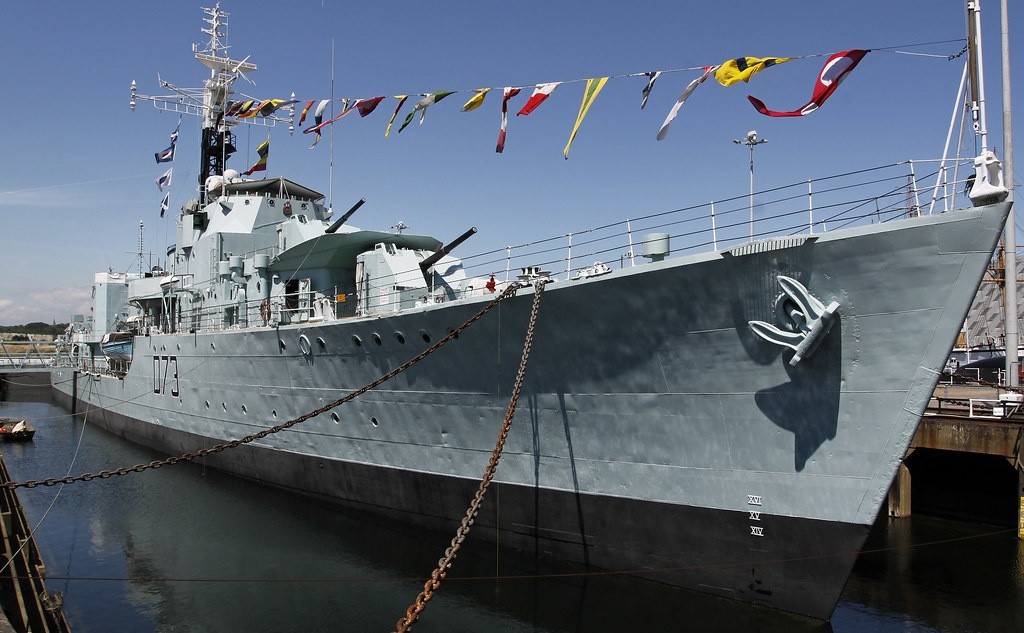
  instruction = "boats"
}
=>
[0,417,36,443]
[51,0,1013,633]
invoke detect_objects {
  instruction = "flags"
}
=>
[155,145,175,164]
[155,168,172,192]
[160,193,169,218]
[225,47,870,158]
[243,138,268,175]
[171,118,181,143]
